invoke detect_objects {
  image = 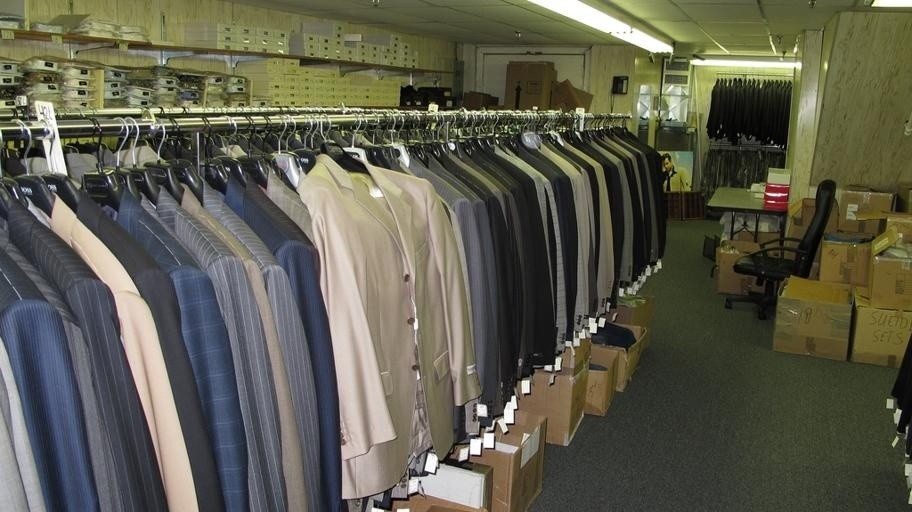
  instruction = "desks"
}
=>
[705,184,788,243]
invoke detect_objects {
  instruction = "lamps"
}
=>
[520,0,675,54]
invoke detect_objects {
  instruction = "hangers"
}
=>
[1,105,627,221]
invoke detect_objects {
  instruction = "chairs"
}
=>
[724,178,838,320]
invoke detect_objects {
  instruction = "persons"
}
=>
[662,154,692,192]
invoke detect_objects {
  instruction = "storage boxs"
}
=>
[463,57,596,114]
[388,293,655,512]
[715,182,912,369]
